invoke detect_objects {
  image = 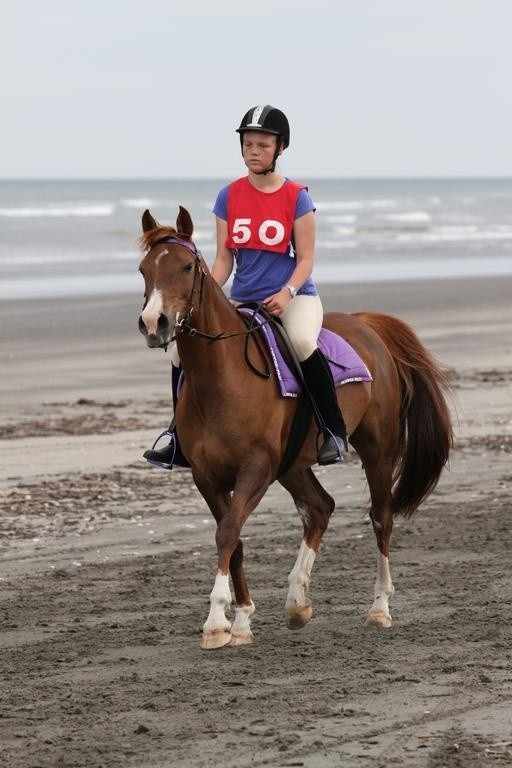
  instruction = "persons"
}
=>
[141,105,347,468]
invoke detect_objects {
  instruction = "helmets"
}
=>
[234,102,290,146]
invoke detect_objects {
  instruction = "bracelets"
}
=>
[281,283,300,300]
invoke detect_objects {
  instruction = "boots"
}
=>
[143,362,192,468]
[299,347,350,467]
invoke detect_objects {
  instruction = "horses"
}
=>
[134,205,459,651]
